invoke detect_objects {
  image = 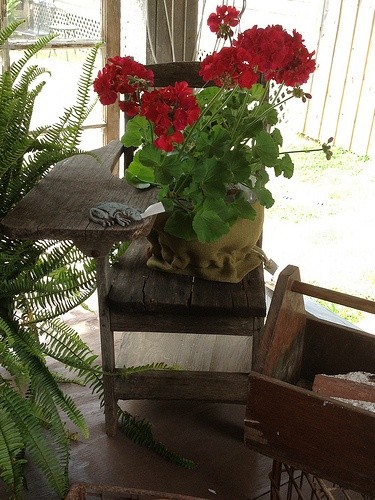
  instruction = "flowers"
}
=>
[94,3,321,241]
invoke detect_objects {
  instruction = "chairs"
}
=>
[4,62,275,430]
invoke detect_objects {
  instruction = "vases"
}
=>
[147,180,264,282]
